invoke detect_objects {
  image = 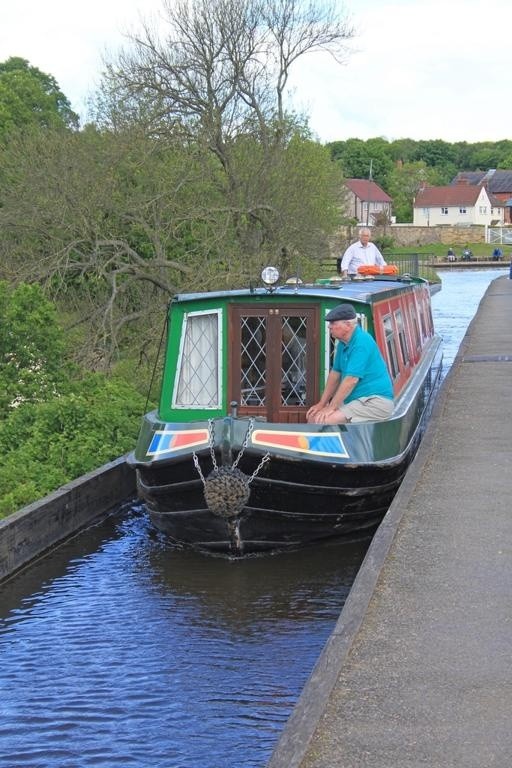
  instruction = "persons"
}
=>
[493,248,502,261]
[448,248,455,262]
[306,304,395,427]
[463,247,473,262]
[341,227,387,279]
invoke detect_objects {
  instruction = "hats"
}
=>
[324,303,356,321]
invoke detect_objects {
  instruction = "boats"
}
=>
[125,267,445,557]
